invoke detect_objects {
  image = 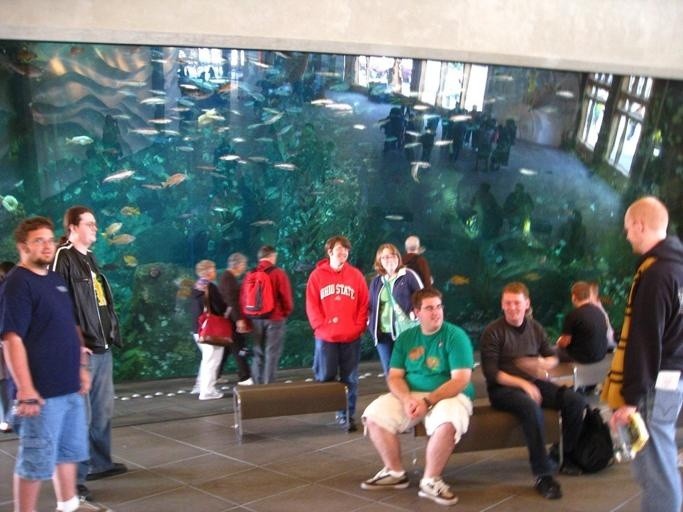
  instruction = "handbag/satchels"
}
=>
[195,312,234,348]
[392,307,420,340]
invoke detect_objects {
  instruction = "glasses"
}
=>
[75,222,96,229]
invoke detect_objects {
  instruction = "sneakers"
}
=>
[235,376,253,386]
[54,498,113,512]
[333,415,357,432]
[190,383,223,401]
[532,473,563,500]
[416,477,459,505]
[359,465,410,491]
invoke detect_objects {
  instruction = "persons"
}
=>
[600,197,683,512]
[503,183,535,236]
[208,67,215,78]
[1,205,129,512]
[379,102,518,178]
[470,183,503,236]
[80,114,123,177]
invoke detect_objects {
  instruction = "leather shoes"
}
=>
[75,484,89,497]
[84,461,130,482]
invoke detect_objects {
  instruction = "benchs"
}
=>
[409,397,568,488]
[227,379,353,445]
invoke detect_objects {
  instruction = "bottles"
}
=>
[610,421,634,462]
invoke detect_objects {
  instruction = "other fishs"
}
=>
[0,41,616,308]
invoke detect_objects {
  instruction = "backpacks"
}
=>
[241,266,280,317]
[564,404,614,475]
[403,254,425,285]
